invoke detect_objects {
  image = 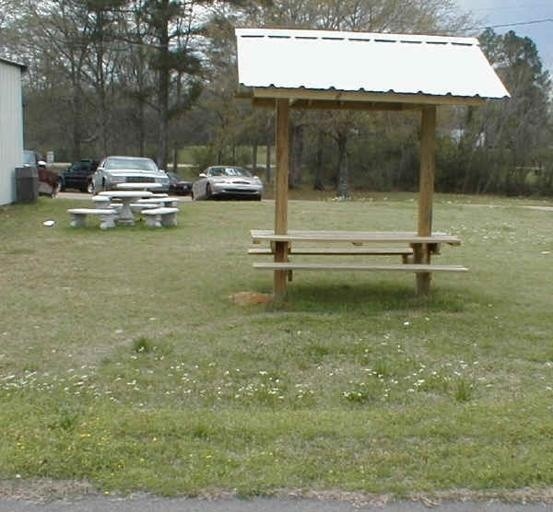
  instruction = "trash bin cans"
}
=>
[15,168,38,203]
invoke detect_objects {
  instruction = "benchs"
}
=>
[246,228,469,298]
[66,182,178,230]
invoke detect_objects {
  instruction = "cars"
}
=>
[24,150,263,201]
